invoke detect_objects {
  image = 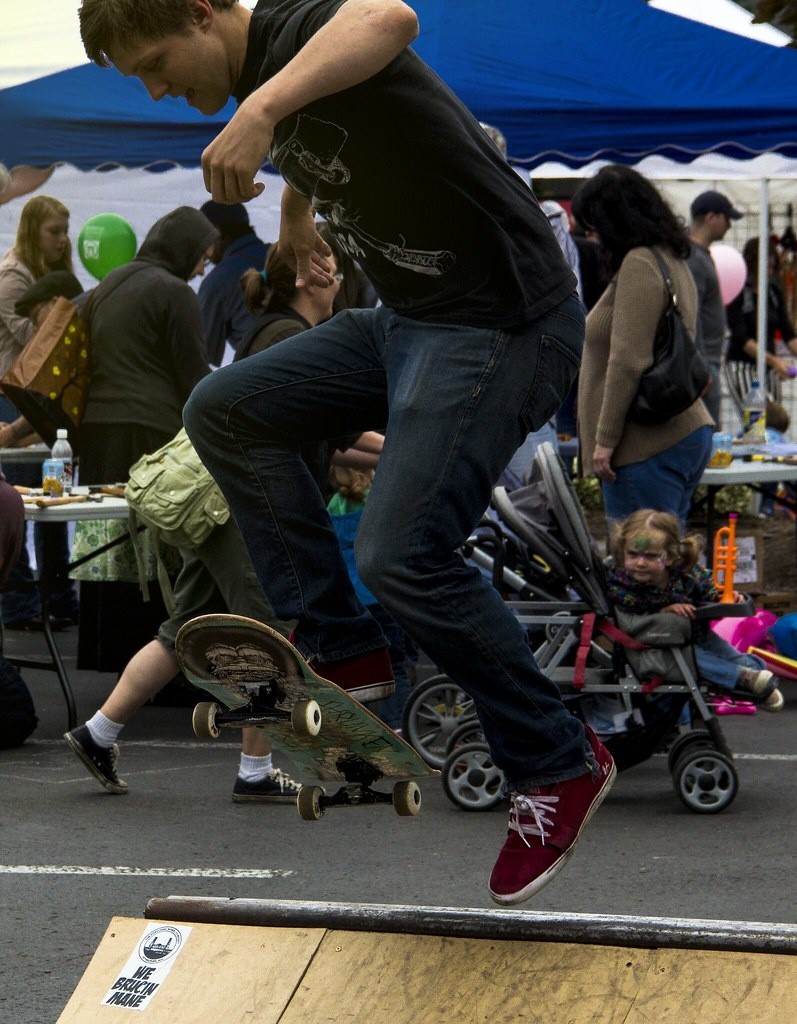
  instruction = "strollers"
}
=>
[402,443,739,816]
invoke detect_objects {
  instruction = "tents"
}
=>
[0,0,797,516]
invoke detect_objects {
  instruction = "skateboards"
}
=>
[174,613,442,819]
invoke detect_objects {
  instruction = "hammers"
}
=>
[36,495,103,509]
[89,486,125,497]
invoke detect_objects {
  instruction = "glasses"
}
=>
[199,251,211,267]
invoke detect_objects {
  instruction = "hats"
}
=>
[691,190,744,221]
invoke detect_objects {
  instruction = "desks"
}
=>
[0,491,157,732]
[555,438,797,574]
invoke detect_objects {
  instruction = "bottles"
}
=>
[743,381,766,444]
[51,429,73,494]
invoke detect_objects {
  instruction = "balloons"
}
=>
[78,213,137,281]
[709,244,747,306]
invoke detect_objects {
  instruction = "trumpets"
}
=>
[713,512,739,606]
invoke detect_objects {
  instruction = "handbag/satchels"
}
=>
[626,246,709,425]
[124,425,231,551]
[1,295,91,452]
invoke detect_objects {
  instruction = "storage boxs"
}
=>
[712,526,797,616]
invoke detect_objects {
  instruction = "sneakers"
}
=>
[487,726,615,907]
[65,725,130,796]
[738,666,785,712]
[306,648,398,703]
[231,770,304,803]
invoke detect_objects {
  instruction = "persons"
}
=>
[535,190,797,538]
[77,1,617,905]
[0,196,387,805]
[609,508,785,714]
